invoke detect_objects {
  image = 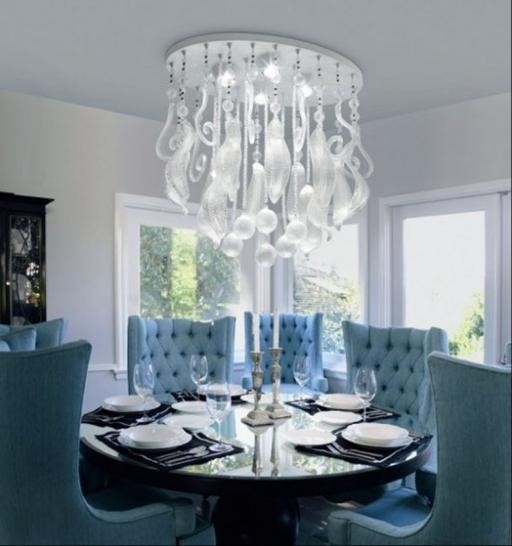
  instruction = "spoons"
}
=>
[159,445,207,462]
[332,442,375,461]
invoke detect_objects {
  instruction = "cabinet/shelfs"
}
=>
[1,192,54,321]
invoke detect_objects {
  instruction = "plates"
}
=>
[163,415,210,431]
[11,228,32,256]
[315,399,371,411]
[12,273,33,301]
[171,401,207,414]
[103,402,162,413]
[314,411,362,426]
[342,428,412,448]
[240,394,273,405]
[117,433,192,450]
[197,385,247,397]
[287,430,337,447]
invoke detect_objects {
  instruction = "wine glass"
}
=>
[190,355,208,400]
[133,364,155,423]
[354,369,377,422]
[206,385,233,452]
[292,356,312,407]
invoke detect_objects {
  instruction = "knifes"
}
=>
[165,450,210,465]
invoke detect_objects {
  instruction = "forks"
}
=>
[326,444,371,463]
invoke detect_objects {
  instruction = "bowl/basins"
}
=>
[319,394,361,408]
[104,396,146,411]
[355,424,409,445]
[129,424,182,447]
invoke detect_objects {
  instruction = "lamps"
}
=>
[154,33,374,270]
